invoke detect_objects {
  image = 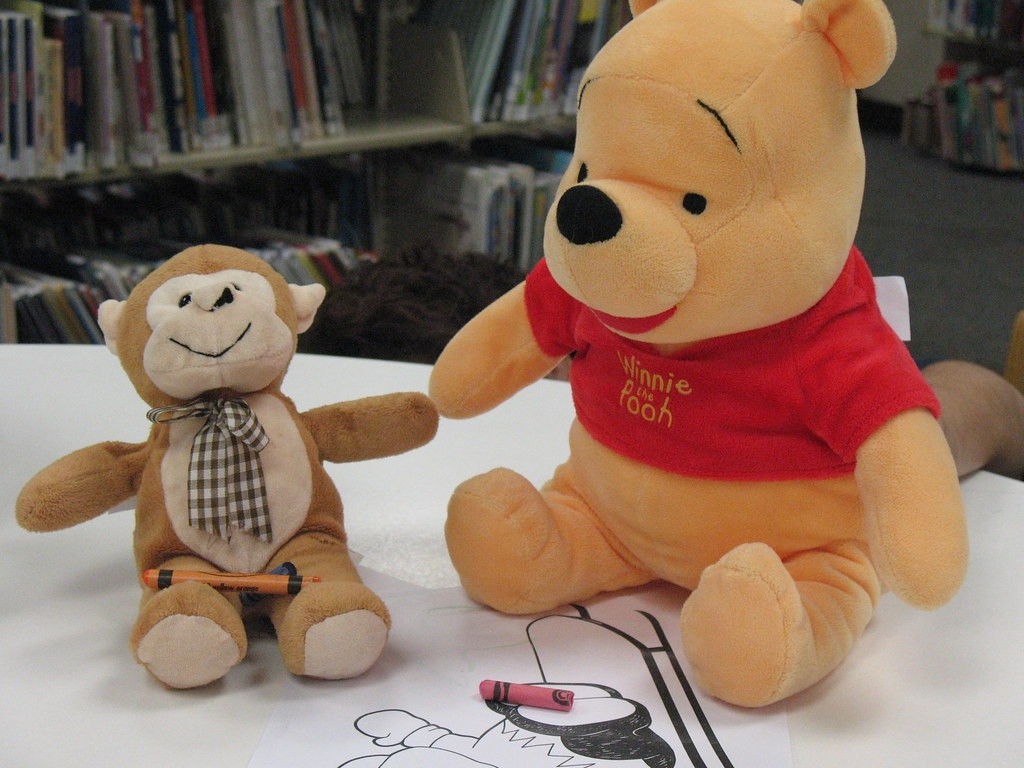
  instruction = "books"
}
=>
[0,0,388,181]
[924,0,1024,170]
[470,0,632,128]
[0,233,377,345]
[418,139,572,273]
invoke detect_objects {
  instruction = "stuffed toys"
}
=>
[430,0,970,709]
[16,244,440,686]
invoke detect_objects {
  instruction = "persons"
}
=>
[920,360,1024,482]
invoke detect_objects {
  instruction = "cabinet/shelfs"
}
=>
[0,0,634,365]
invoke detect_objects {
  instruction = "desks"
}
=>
[0,342,1024,768]
